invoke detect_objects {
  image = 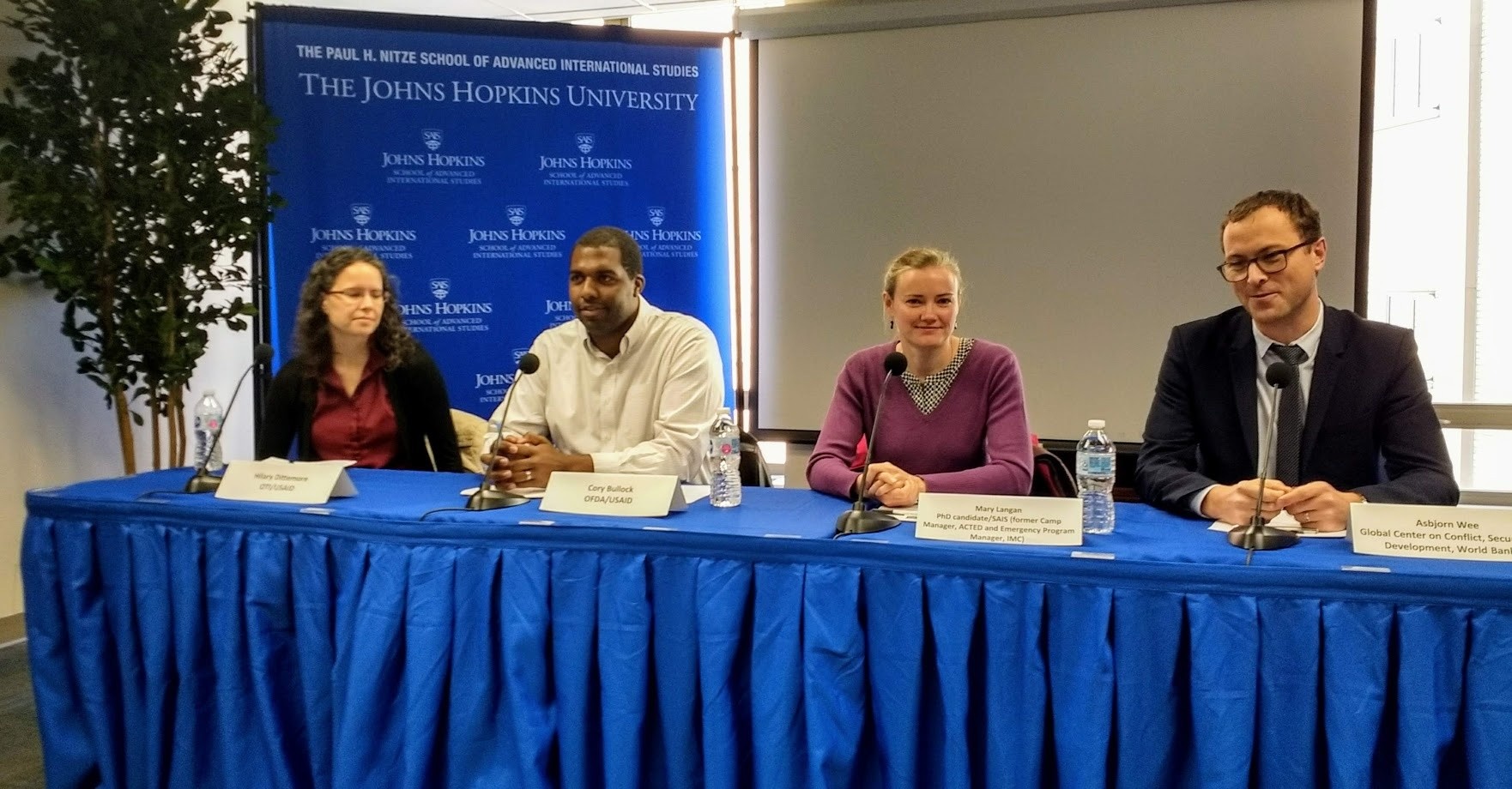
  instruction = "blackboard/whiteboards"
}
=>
[732,0,1377,455]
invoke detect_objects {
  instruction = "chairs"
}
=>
[1030,432,1078,498]
[424,409,489,474]
[738,428,774,488]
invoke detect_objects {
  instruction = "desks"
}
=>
[19,464,1512,789]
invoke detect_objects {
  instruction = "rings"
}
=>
[1306,511,1310,522]
[525,471,534,480]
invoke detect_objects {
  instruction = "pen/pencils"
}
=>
[497,424,504,441]
[872,509,917,515]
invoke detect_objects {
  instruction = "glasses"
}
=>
[325,286,392,305]
[1219,236,1318,282]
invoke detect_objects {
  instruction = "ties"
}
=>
[1270,343,1306,487]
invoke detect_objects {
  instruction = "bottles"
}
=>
[1077,420,1115,534]
[194,391,223,472]
[709,408,741,507]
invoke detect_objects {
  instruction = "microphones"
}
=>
[185,343,274,493]
[835,352,908,532]
[466,354,540,510]
[1228,360,1299,551]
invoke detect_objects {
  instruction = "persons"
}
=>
[1137,191,1459,530]
[241,245,464,473]
[805,247,1034,509]
[472,227,726,492]
